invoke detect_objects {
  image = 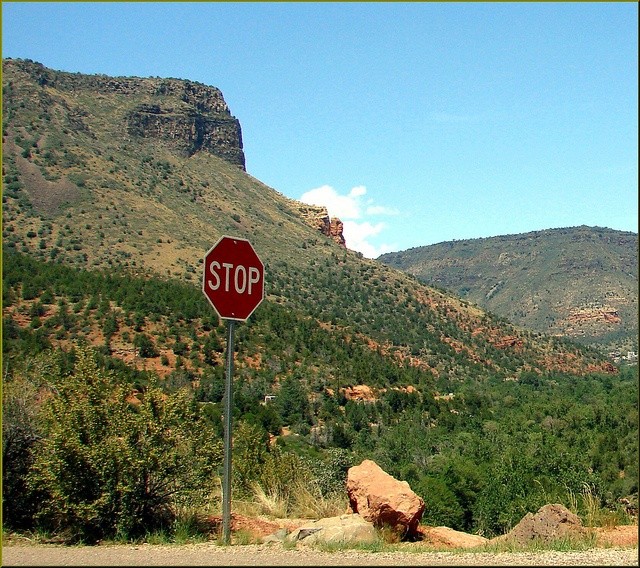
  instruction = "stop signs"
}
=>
[202,235,265,321]
